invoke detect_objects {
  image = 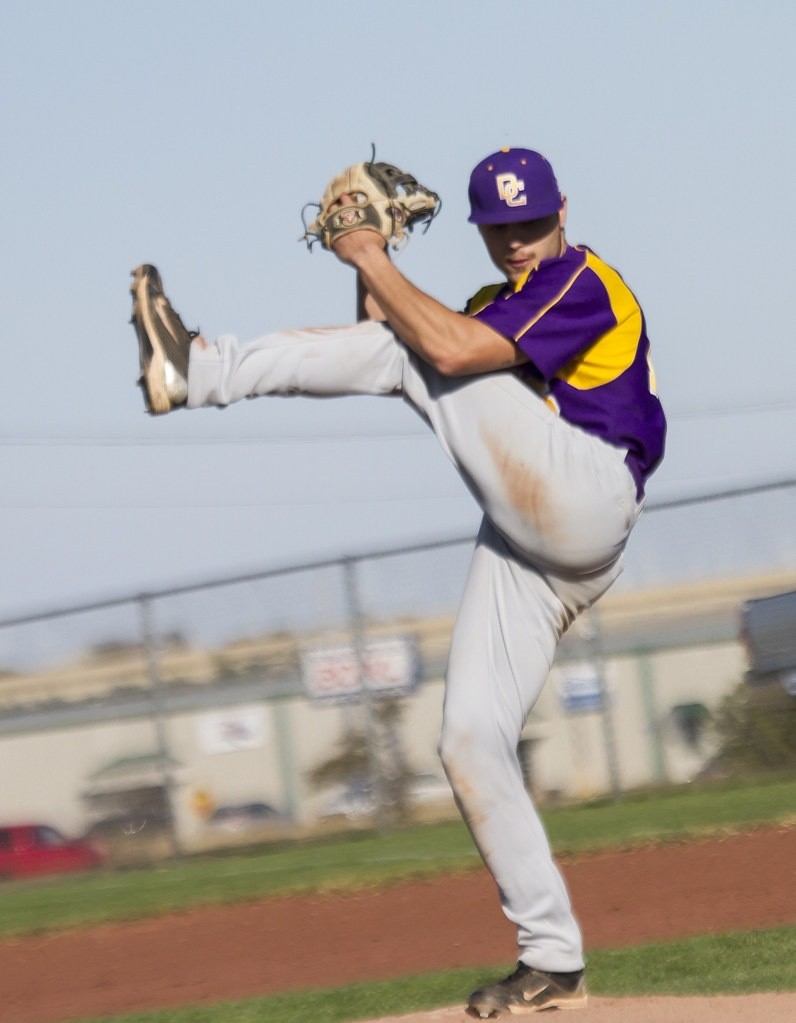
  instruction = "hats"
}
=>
[468,147,564,225]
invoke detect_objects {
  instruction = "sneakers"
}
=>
[128,263,200,417]
[467,961,588,1019]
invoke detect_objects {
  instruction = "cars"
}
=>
[1,825,102,878]
[207,803,288,832]
[86,814,173,848]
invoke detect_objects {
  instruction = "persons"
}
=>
[128,144,666,1020]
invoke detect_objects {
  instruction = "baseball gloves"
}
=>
[312,161,438,252]
